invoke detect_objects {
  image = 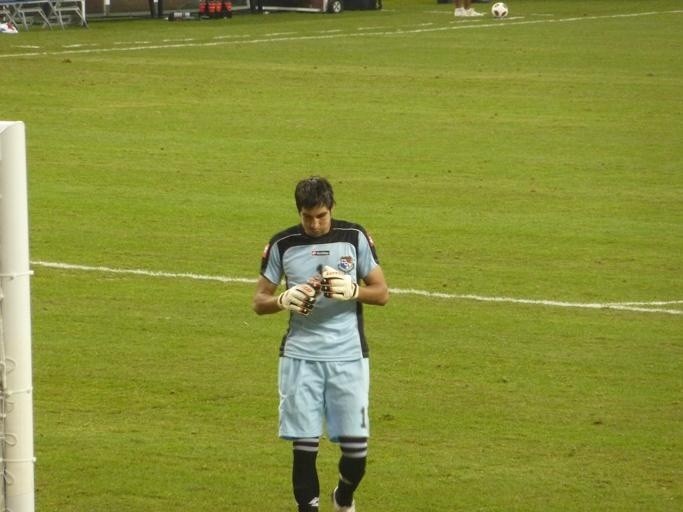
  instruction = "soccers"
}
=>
[491,2,509,19]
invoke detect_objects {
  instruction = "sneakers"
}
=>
[455,8,483,16]
[332,486,355,512]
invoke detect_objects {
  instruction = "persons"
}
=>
[253,177,389,512]
[454,0,486,16]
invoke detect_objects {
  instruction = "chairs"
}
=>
[0,0,90,30]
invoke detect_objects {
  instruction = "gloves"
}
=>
[314,264,359,300]
[277,282,320,316]
